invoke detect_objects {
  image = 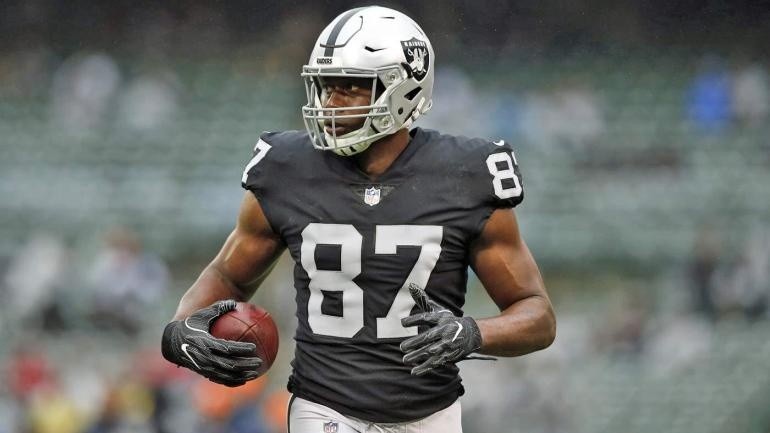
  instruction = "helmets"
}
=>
[300,5,434,156]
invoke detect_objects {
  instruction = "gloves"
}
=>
[399,283,484,377]
[161,300,262,387]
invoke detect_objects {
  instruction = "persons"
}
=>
[161,5,556,433]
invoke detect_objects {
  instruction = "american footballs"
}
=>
[208,301,279,380]
